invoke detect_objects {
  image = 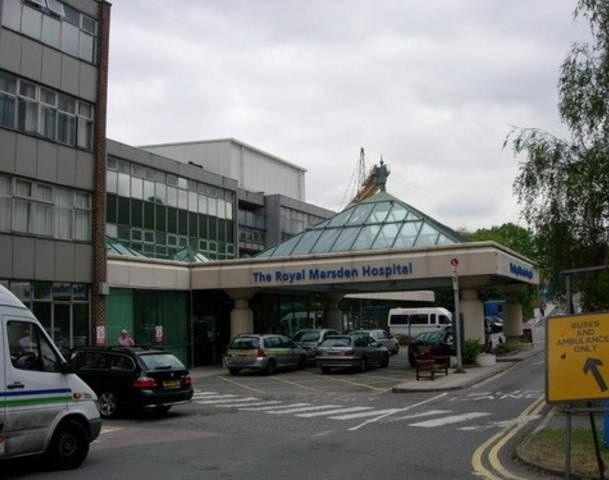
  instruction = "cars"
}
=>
[223,328,399,376]
[409,332,450,368]
[59,344,194,418]
[485,316,507,350]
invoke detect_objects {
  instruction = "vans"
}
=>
[0,282,101,469]
[387,306,455,346]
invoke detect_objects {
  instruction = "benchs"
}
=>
[409,342,450,380]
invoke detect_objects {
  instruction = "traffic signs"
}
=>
[545,312,608,404]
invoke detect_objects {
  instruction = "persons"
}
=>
[116,329,135,347]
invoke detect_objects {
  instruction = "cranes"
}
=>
[340,147,390,210]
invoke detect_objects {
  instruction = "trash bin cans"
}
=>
[523,329,532,343]
